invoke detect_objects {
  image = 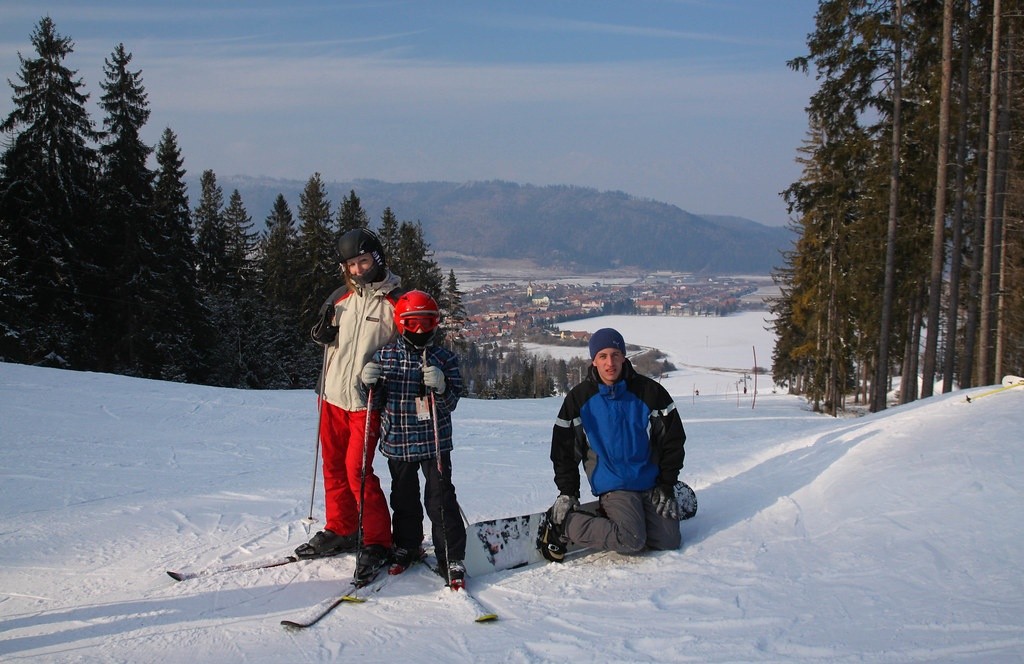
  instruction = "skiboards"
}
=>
[342,536,499,623]
[167,549,363,627]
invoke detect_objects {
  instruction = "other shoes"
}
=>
[354,545,394,579]
[388,545,420,575]
[294,530,347,556]
[441,560,467,592]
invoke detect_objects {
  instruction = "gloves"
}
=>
[362,363,382,387]
[550,495,580,524]
[652,487,678,519]
[314,305,340,344]
[423,366,444,392]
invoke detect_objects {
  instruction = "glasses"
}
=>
[395,315,438,332]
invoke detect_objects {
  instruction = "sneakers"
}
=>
[542,507,567,563]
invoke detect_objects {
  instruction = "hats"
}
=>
[589,328,626,362]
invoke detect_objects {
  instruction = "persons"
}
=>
[356,290,468,583]
[535,328,686,563]
[295,227,405,582]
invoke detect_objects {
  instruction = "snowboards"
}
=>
[458,479,698,577]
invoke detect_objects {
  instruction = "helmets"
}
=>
[338,228,384,284]
[394,290,438,334]
